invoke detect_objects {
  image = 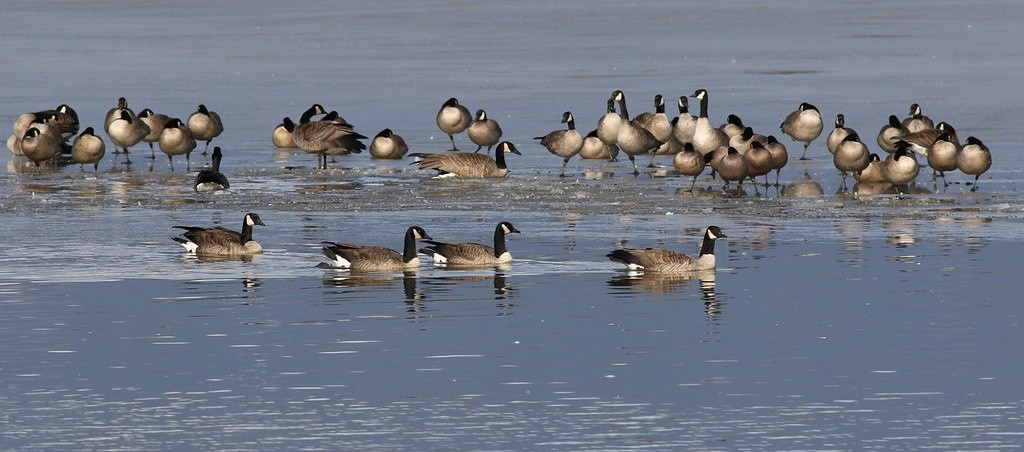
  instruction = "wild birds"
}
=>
[605,226,728,272]
[321,226,433,269]
[7,89,992,193]
[418,221,520,266]
[171,212,266,256]
